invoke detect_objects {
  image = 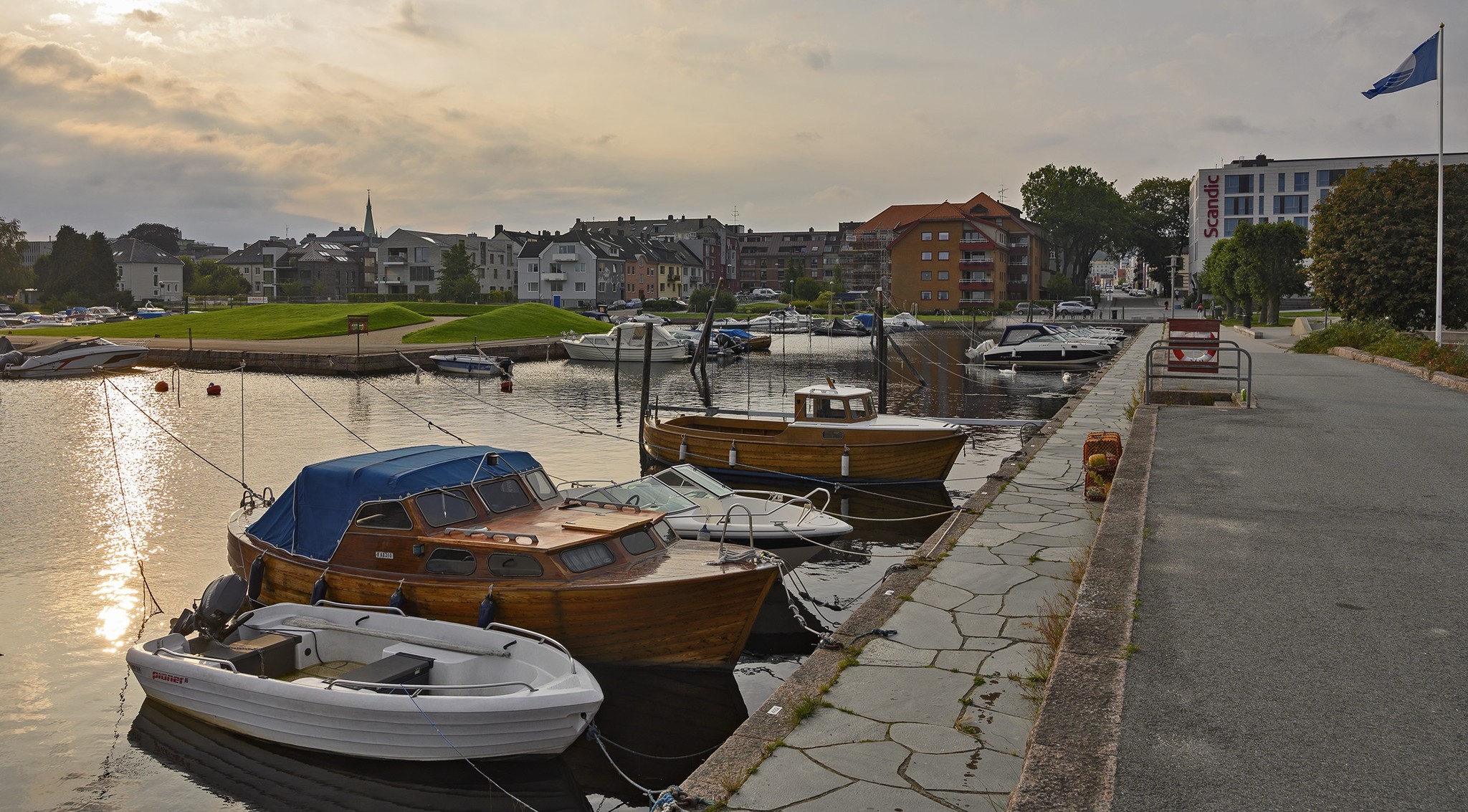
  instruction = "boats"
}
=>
[135,300,173,318]
[810,317,872,337]
[0,335,149,380]
[558,321,770,367]
[581,311,672,325]
[982,321,1126,373]
[228,442,783,672]
[124,698,594,812]
[559,466,857,579]
[428,354,513,376]
[127,600,606,760]
[883,312,933,332]
[748,307,824,334]
[0,304,131,327]
[641,383,978,493]
[843,315,891,335]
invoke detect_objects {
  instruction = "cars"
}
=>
[607,299,626,310]
[1094,283,1146,297]
[735,292,753,301]
[644,295,692,310]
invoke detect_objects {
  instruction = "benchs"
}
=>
[198,630,302,678]
[1131,315,1168,320]
[1233,324,1264,339]
[327,651,435,696]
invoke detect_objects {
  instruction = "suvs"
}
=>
[1014,302,1051,315]
[626,298,642,309]
[1056,296,1095,315]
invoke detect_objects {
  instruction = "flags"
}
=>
[1361,29,1440,99]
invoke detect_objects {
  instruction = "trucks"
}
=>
[753,288,786,300]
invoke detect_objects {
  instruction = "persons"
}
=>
[1114,284,1118,289]
[1148,288,1152,295]
[1175,289,1179,300]
[588,299,593,311]
[1164,299,1169,312]
[788,302,792,308]
[1153,288,1157,296]
[1197,303,1203,319]
[1090,301,1094,307]
[116,306,122,316]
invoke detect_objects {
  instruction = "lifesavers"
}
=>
[1172,331,1217,362]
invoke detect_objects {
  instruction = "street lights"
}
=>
[261,280,265,303]
[727,264,733,289]
[159,281,164,299]
[829,281,834,316]
[790,280,794,302]
